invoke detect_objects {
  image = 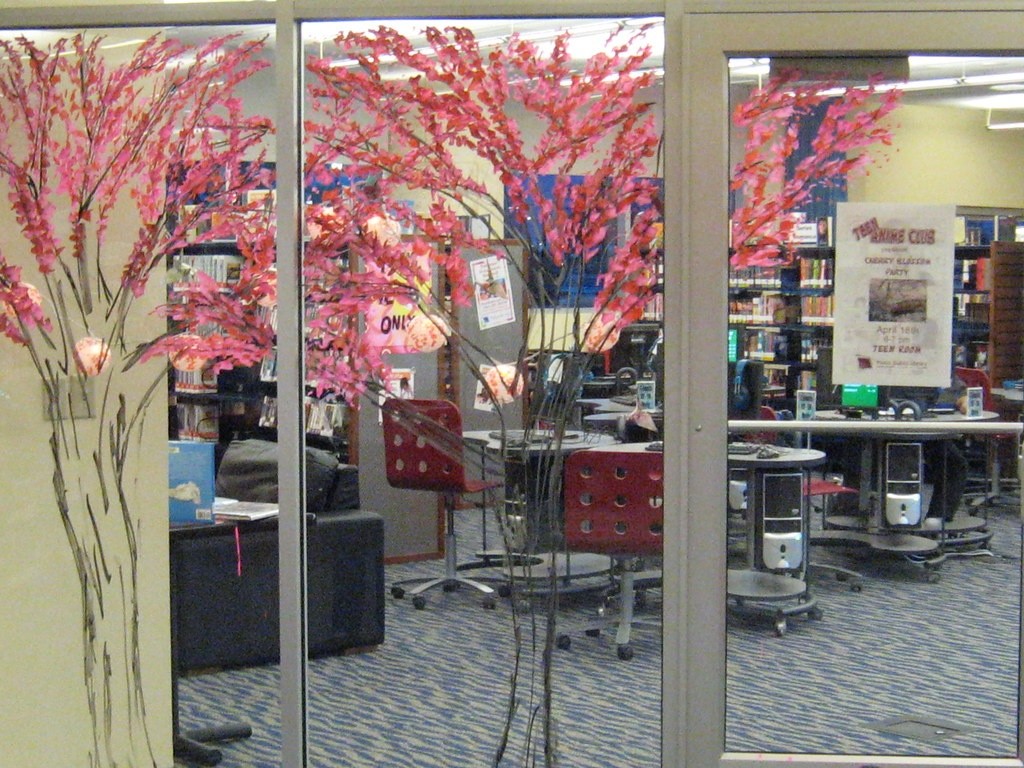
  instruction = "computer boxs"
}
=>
[747,468,804,574]
[503,459,566,553]
[884,442,923,529]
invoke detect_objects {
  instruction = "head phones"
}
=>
[544,353,583,403]
[729,360,752,411]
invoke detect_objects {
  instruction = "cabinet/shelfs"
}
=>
[165,235,529,564]
[611,242,1024,491]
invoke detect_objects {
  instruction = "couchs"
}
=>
[170,464,384,677]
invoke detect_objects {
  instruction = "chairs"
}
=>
[553,450,665,664]
[382,398,512,610]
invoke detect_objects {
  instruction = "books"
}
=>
[954,220,992,371]
[1015,379,1024,388]
[172,189,347,443]
[728,218,836,396]
[214,501,279,520]
[639,222,663,322]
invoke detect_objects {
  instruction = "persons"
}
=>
[930,377,970,522]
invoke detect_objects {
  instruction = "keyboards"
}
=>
[489,429,549,444]
[645,441,760,455]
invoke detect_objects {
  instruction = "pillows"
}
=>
[216,438,340,511]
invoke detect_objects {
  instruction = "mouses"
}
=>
[756,448,779,459]
[507,439,530,447]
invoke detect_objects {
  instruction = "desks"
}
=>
[461,429,622,598]
[591,441,825,637]
[807,410,1001,583]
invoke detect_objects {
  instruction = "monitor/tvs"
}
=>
[727,362,765,420]
[530,352,588,423]
[841,384,879,411]
